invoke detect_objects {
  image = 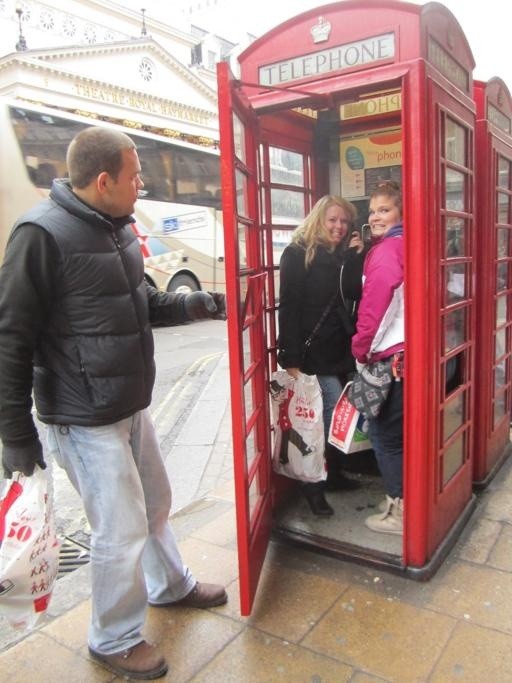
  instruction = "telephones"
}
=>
[343,224,357,260]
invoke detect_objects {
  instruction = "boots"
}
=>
[365,493,404,537]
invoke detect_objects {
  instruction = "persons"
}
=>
[351,182,403,535]
[0,125,243,679]
[276,196,364,518]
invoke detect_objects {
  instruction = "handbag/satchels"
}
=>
[328,378,374,455]
[0,456,59,657]
[278,329,320,374]
[347,359,393,421]
[269,370,328,484]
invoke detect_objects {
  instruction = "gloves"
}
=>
[185,289,227,321]
[2,437,46,479]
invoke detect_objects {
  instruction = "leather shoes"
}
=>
[148,581,227,608]
[89,635,168,680]
[301,485,334,515]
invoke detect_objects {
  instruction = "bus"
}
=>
[0,96,305,321]
